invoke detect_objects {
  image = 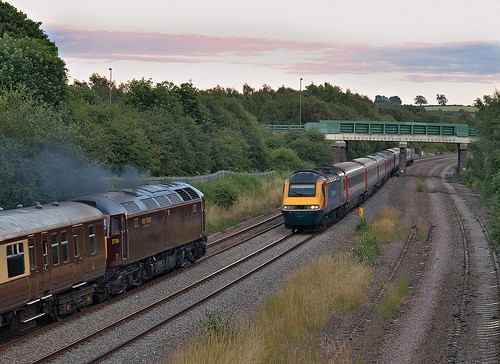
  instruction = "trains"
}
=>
[1,181,208,343]
[279,142,416,235]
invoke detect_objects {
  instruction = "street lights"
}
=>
[300,77,304,132]
[108,67,112,106]
